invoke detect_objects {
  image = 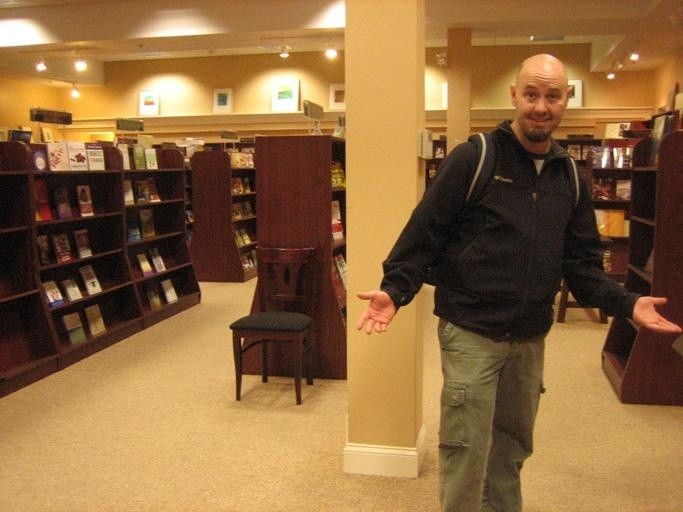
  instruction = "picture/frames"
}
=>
[272,78,300,112]
[329,83,345,110]
[566,79,583,108]
[139,90,160,116]
[213,87,233,114]
[651,113,668,140]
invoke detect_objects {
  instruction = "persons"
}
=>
[358,52,682,510]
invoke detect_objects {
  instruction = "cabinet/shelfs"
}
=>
[188,136,258,283]
[425,139,447,188]
[242,137,348,378]
[2,135,202,399]
[553,128,683,405]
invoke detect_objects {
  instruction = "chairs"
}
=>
[228,244,318,403]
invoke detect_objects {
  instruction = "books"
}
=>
[428,143,444,182]
[564,143,634,274]
[182,146,258,271]
[648,115,676,165]
[116,137,178,311]
[331,157,347,322]
[10,125,106,346]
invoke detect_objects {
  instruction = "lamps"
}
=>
[279,46,289,58]
[436,51,447,66]
[606,50,639,80]
